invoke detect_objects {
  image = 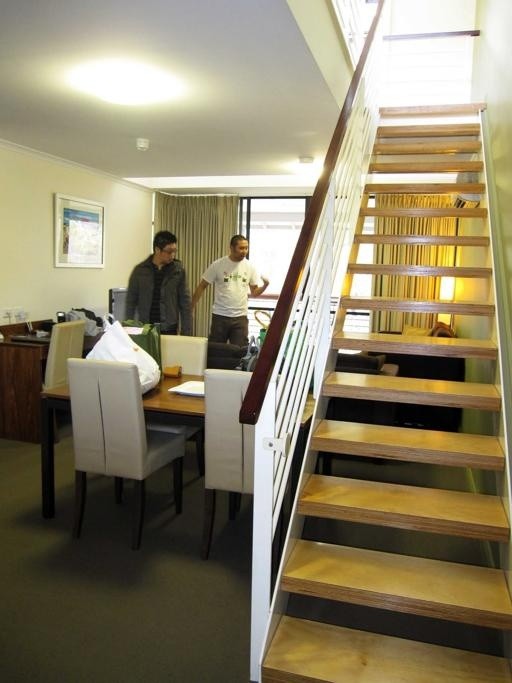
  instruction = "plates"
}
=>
[168,381,205,397]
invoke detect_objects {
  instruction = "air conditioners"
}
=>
[451,172,480,209]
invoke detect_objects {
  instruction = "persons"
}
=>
[187,233,270,348]
[124,230,194,337]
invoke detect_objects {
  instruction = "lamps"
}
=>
[438,276,455,325]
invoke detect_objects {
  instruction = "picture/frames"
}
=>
[53,193,107,269]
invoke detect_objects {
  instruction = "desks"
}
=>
[0,320,105,445]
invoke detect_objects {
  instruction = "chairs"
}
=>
[42,320,85,443]
[145,333,209,477]
[200,368,294,560]
[326,354,399,462]
[67,359,186,550]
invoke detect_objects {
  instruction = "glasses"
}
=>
[162,248,177,254]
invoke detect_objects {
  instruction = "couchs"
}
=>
[368,322,465,433]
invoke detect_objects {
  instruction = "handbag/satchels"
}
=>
[121,319,161,384]
[84,311,161,394]
